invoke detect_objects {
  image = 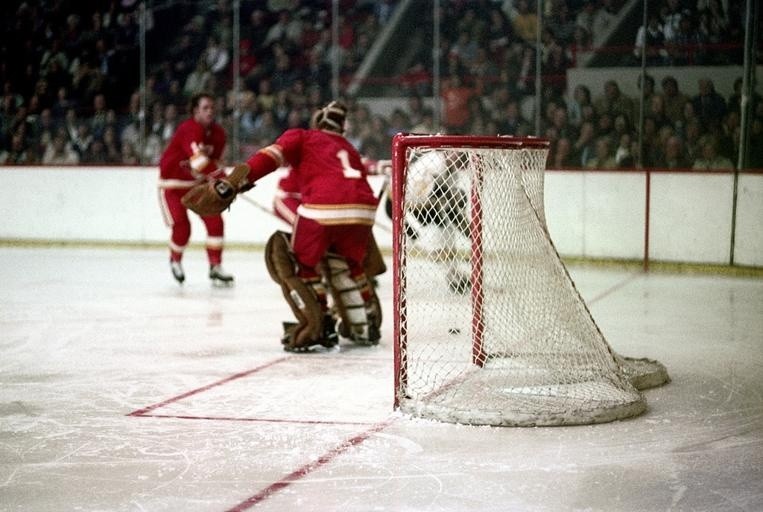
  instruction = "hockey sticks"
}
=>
[371,221,511,293]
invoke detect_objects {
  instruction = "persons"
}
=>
[1,1,763,170]
[157,92,233,285]
[180,99,473,354]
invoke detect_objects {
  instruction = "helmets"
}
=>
[312,101,347,133]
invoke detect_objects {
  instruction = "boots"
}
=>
[170,260,184,281]
[209,265,232,281]
